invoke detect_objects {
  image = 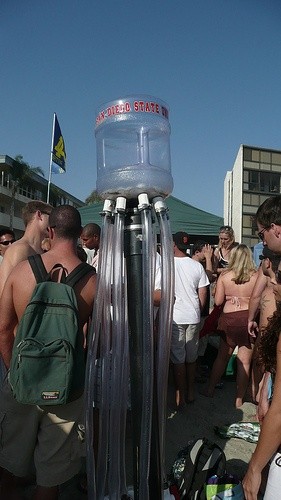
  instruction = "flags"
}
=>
[51,113,67,175]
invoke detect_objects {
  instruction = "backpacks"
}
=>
[169,437,225,500]
[7,254,97,406]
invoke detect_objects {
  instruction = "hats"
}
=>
[173,231,191,250]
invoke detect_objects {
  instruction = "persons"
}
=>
[254,195,281,253]
[0,204,114,500]
[242,259,281,500]
[0,201,54,338]
[0,223,281,427]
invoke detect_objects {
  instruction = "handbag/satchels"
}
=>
[203,474,246,500]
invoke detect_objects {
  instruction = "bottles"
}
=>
[94,94,175,205]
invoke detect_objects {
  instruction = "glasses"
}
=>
[258,255,273,260]
[220,226,232,231]
[275,271,281,285]
[257,225,272,239]
[0,239,16,245]
